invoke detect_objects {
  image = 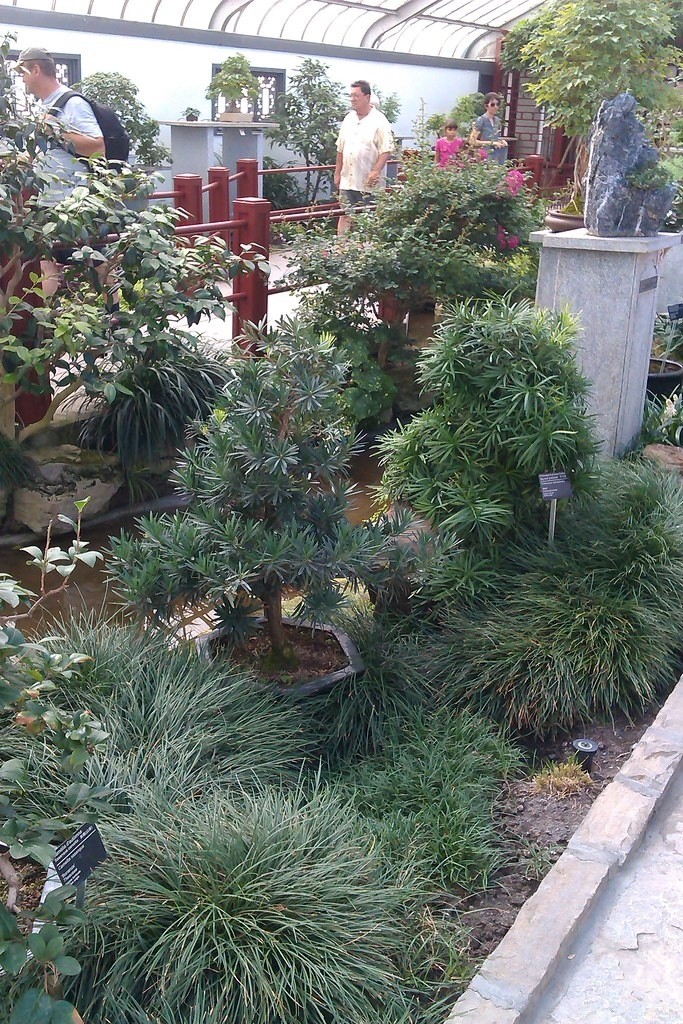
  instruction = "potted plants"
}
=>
[100,316,367,703]
[206,53,261,123]
[648,304,683,406]
[520,0,682,229]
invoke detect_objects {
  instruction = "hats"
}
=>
[11,46,54,74]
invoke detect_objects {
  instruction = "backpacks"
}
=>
[48,91,129,174]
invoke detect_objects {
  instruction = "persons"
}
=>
[434,120,464,166]
[13,48,121,313]
[468,92,508,161]
[334,80,391,237]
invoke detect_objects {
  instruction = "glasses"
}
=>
[486,102,500,107]
